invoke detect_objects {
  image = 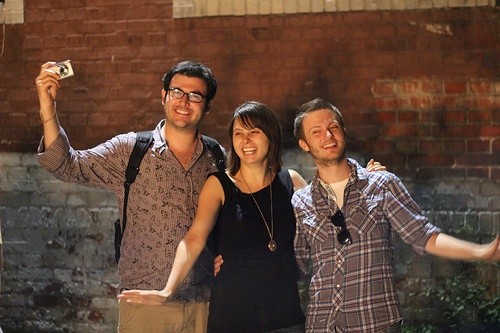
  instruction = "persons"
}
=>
[213,97,500,333]
[117,100,386,333]
[36,61,227,333]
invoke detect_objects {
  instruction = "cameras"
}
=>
[47,60,74,80]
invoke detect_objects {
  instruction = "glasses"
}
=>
[167,88,207,103]
[331,210,352,244]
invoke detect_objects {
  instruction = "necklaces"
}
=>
[241,168,277,251]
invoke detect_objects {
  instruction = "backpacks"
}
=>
[113,131,224,261]
